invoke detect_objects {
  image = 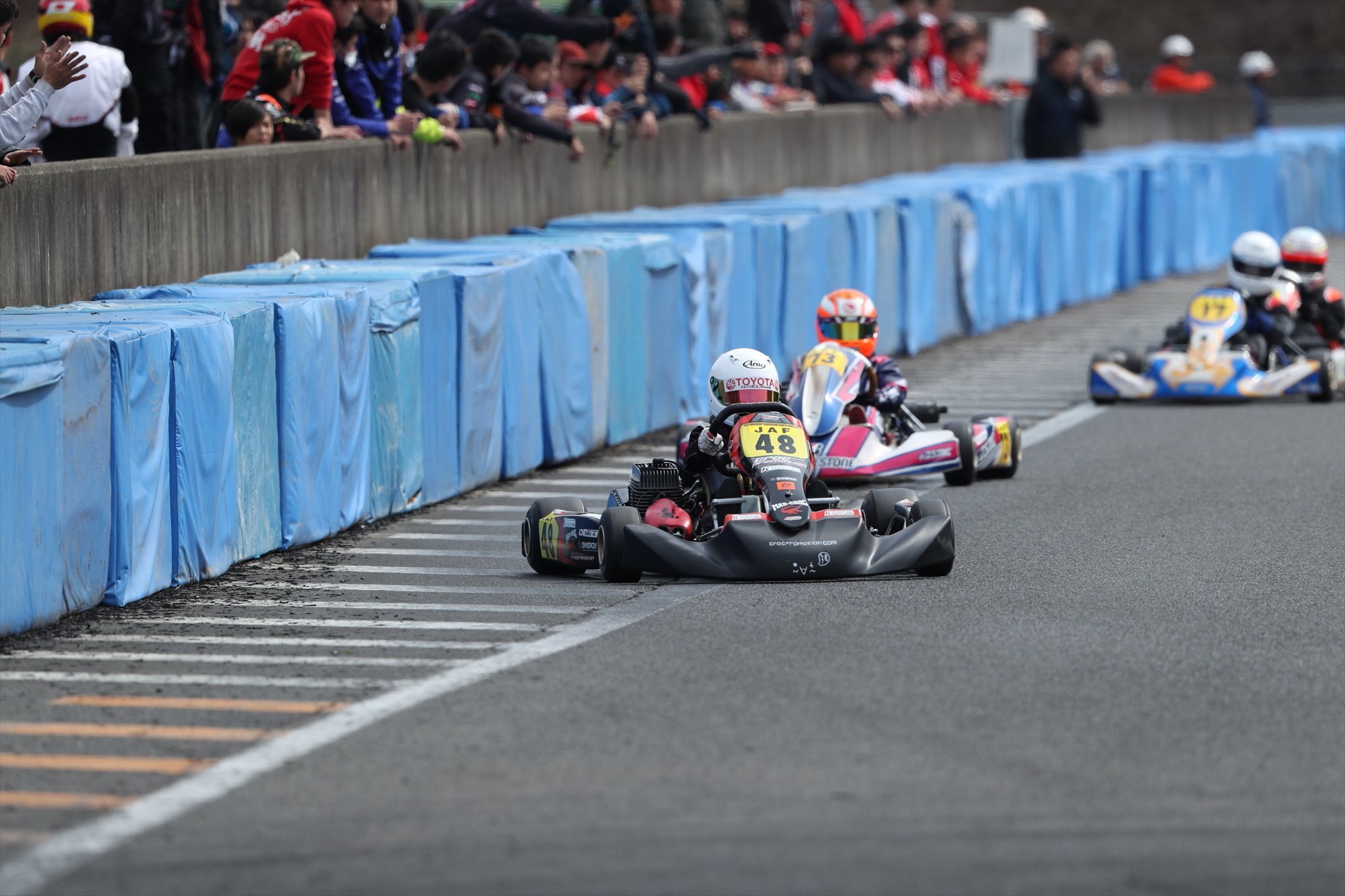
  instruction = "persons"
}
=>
[0,0,1133,189]
[1150,34,1214,94]
[1236,51,1278,125]
[1163,225,1345,371]
[786,288,907,447]
[683,348,830,533]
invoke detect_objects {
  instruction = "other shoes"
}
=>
[1246,335,1267,368]
[806,478,830,512]
[715,477,744,531]
[848,404,866,425]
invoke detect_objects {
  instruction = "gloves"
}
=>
[866,388,891,410]
[698,427,724,455]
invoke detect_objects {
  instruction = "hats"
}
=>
[556,41,593,69]
[257,38,316,73]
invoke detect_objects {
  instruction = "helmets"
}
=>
[1160,34,1196,61]
[1240,50,1274,81]
[816,288,879,356]
[1227,231,1281,296]
[1281,226,1330,292]
[708,348,783,426]
[36,0,95,39]
[1009,6,1051,33]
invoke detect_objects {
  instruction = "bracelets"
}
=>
[29,68,41,83]
